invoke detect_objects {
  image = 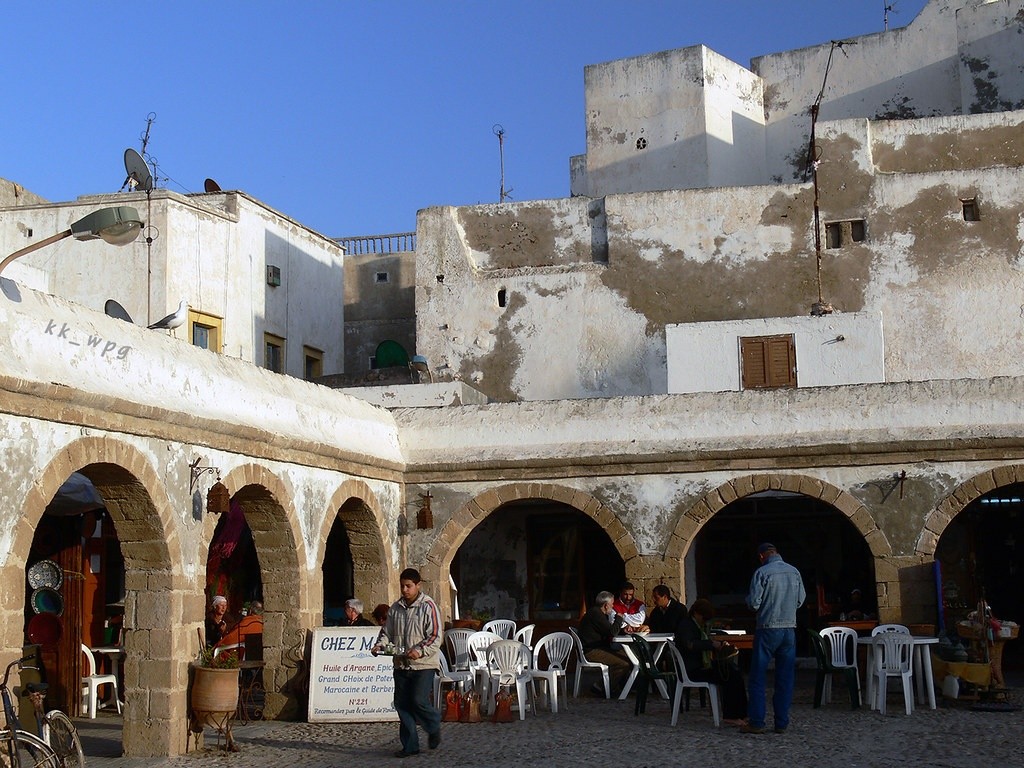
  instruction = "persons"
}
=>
[675,598,750,727]
[251,595,263,608]
[740,543,807,733]
[608,582,653,693]
[631,585,688,665]
[371,604,391,625]
[205,596,227,659]
[576,591,631,699]
[371,568,443,758]
[345,599,375,626]
[841,589,861,618]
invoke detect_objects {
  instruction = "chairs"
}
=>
[81,643,121,720]
[434,620,914,727]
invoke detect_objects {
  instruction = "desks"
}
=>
[475,646,532,711]
[856,636,939,709]
[709,634,754,665]
[827,621,880,632]
[91,648,125,709]
[612,633,675,701]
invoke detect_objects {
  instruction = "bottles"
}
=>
[385,641,406,655]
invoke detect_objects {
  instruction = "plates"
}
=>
[28,559,63,590]
[632,631,649,637]
[31,586,65,617]
[27,612,64,651]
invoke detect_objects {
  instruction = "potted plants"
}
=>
[185,642,241,729]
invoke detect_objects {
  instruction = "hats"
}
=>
[211,596,227,606]
[758,543,776,552]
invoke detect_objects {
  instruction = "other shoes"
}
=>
[740,724,763,734]
[428,728,441,749]
[775,726,785,734]
[395,747,420,758]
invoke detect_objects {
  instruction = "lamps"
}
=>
[0,206,141,275]
[412,355,433,383]
[189,457,232,514]
[415,490,434,531]
